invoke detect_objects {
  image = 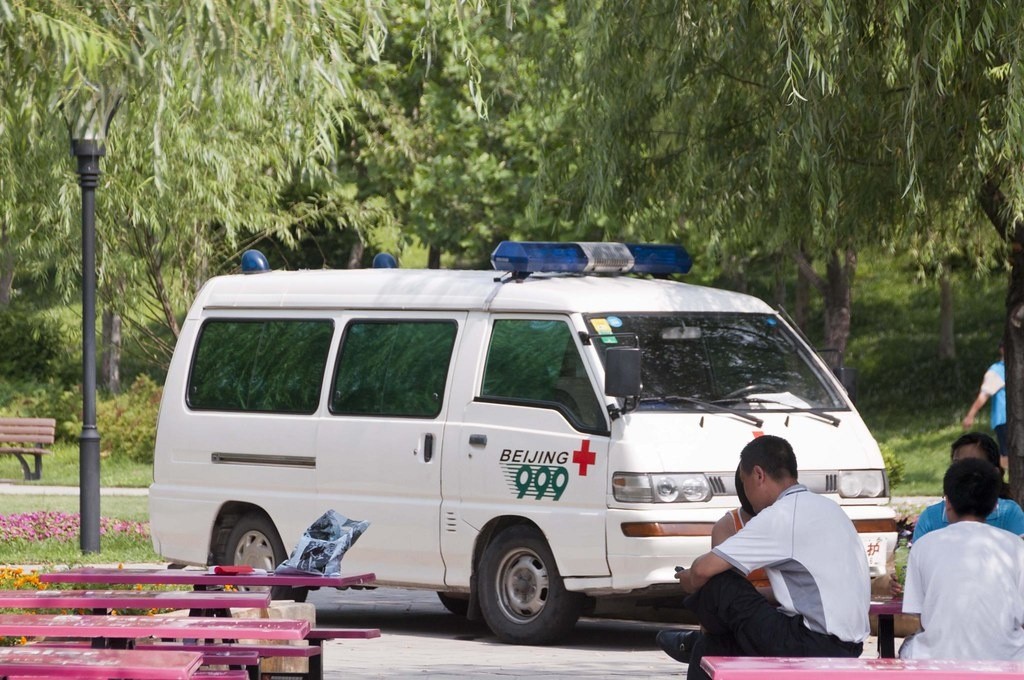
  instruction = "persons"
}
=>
[711,462,778,606]
[888,431,1024,596]
[962,346,1009,468]
[898,456,1024,662]
[655,435,871,680]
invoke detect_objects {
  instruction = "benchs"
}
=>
[0,417,56,480]
[7,628,380,680]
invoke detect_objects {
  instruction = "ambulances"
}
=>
[147,243,899,645]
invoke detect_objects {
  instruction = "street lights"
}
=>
[58,85,125,557]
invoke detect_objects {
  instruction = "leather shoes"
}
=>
[656,629,702,664]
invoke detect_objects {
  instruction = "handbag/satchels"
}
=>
[273,509,370,575]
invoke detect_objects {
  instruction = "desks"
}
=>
[700,656,1024,680]
[869,598,904,659]
[0,614,311,650]
[0,590,271,649]
[40,567,375,671]
[0,646,203,680]
